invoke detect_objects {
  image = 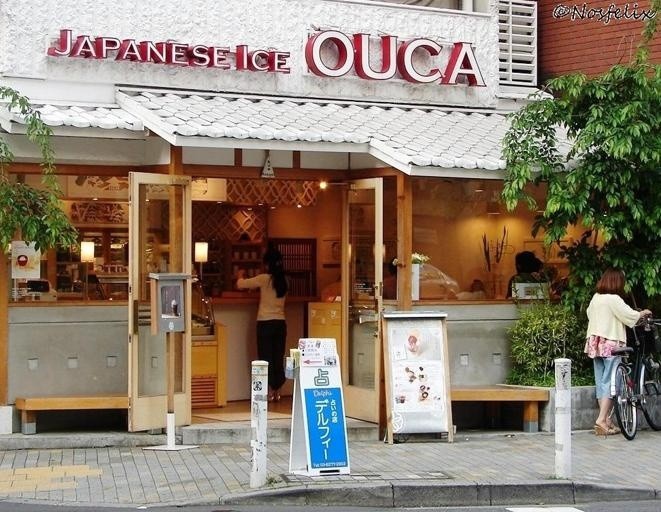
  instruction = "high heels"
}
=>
[268,390,281,402]
[593,419,621,436]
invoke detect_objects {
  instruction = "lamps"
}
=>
[81,241,96,296]
[194,241,209,277]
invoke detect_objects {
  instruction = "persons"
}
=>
[236,248,289,402]
[506,250,552,300]
[583,268,653,435]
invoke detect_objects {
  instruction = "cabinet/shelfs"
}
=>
[192,262,228,409]
[226,238,265,292]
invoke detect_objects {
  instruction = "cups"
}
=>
[234,251,260,278]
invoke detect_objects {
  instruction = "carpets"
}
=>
[193,411,291,421]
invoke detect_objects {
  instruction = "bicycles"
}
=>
[608,317,661,440]
